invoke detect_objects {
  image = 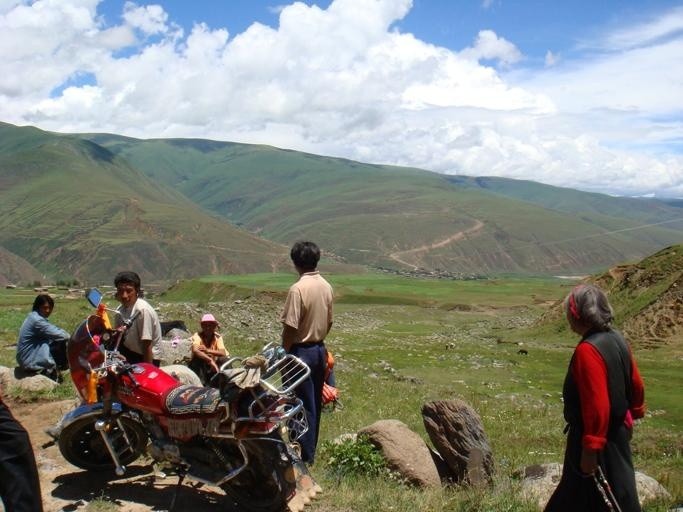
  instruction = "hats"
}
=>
[201,314,217,326]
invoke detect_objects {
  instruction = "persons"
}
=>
[0,400,43,512]
[187,314,232,387]
[16,294,71,385]
[279,241,333,469]
[542,284,645,512]
[112,270,163,368]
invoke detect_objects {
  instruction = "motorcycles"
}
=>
[41,289,321,512]
